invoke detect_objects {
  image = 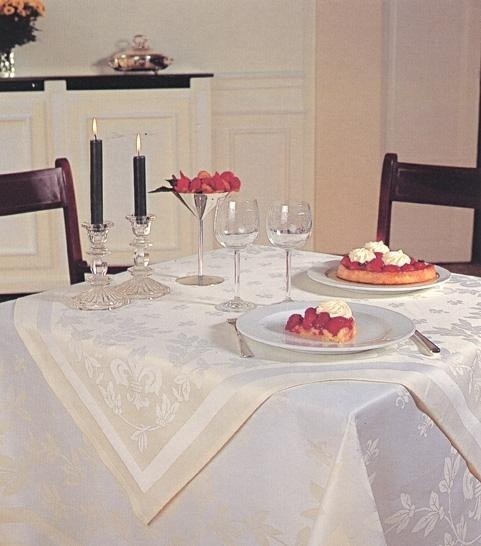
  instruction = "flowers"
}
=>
[0,0,46,70]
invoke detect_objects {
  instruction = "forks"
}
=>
[226,316,255,359]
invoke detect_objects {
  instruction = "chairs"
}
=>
[1,158,133,304]
[376,153,481,275]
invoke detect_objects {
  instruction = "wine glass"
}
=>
[170,188,230,288]
[264,200,313,300]
[214,197,261,314]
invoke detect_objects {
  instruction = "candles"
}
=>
[133,132,148,223]
[88,117,104,225]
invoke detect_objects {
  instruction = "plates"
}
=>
[306,258,453,294]
[234,300,416,356]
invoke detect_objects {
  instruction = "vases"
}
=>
[0,50,17,77]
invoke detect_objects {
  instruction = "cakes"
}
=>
[336,240,437,285]
[284,300,356,343]
